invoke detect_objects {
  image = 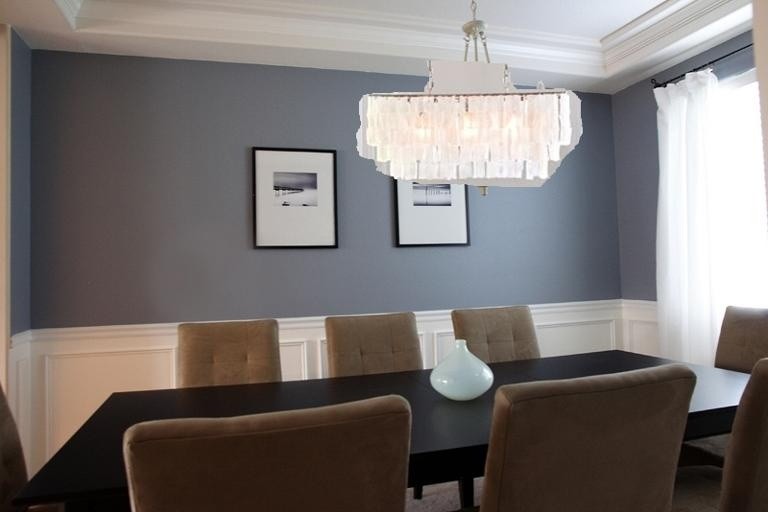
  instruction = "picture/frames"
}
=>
[251,145,340,251]
[392,175,472,249]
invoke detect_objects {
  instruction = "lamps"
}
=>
[354,1,584,197]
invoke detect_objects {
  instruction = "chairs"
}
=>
[450,306,540,362]
[176,319,284,391]
[123,393,412,512]
[1,376,58,512]
[718,359,767,512]
[686,306,768,466]
[481,363,697,512]
[325,312,423,377]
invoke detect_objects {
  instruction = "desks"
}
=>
[9,351,698,512]
